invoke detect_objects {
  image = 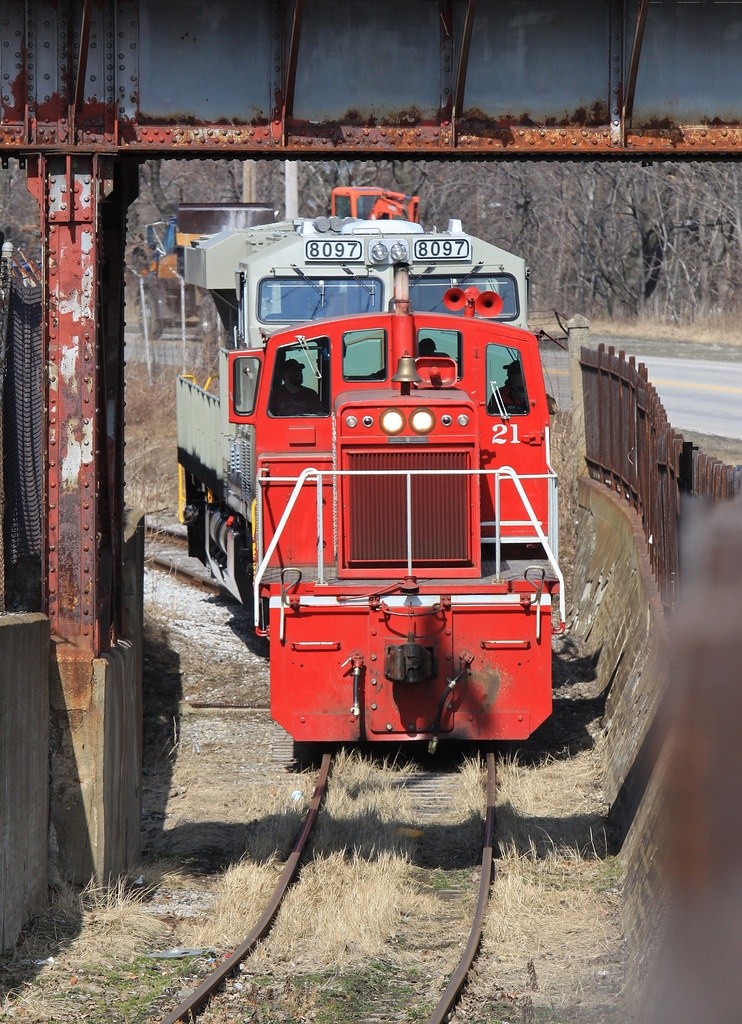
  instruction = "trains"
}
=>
[172,183,567,763]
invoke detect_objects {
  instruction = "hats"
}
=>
[280,359,305,374]
[503,361,520,369]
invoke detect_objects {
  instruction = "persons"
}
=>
[418,338,437,356]
[272,359,317,400]
[488,360,529,414]
